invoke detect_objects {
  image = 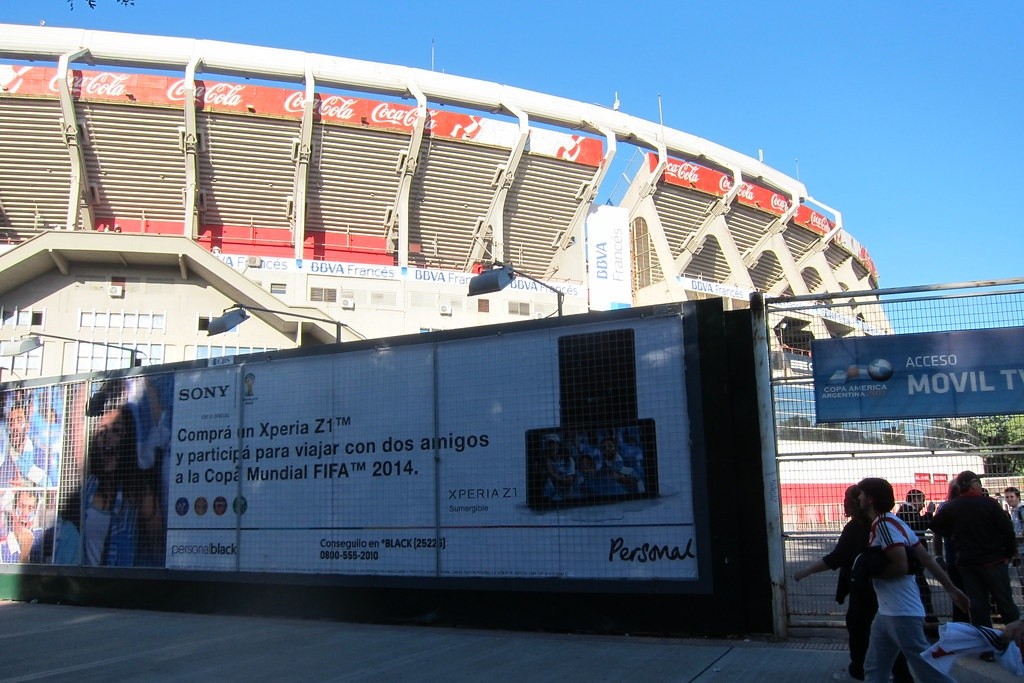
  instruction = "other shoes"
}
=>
[832,671,865,683]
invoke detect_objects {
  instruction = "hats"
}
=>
[957,471,987,485]
[546,434,559,442]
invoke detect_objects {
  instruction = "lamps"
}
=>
[206,303,347,347]
[0,331,137,370]
[466,259,564,318]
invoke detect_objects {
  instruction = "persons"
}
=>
[0,375,172,569]
[932,480,1006,624]
[1005,487,1024,602]
[1000,612,1024,647]
[929,471,1021,629]
[540,428,645,501]
[847,478,971,683]
[795,485,915,683]
[896,489,940,645]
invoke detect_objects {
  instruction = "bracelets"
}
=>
[934,555,943,560]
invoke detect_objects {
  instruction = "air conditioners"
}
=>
[248,256,261,267]
[108,286,122,296]
[341,298,354,308]
[440,305,451,315]
